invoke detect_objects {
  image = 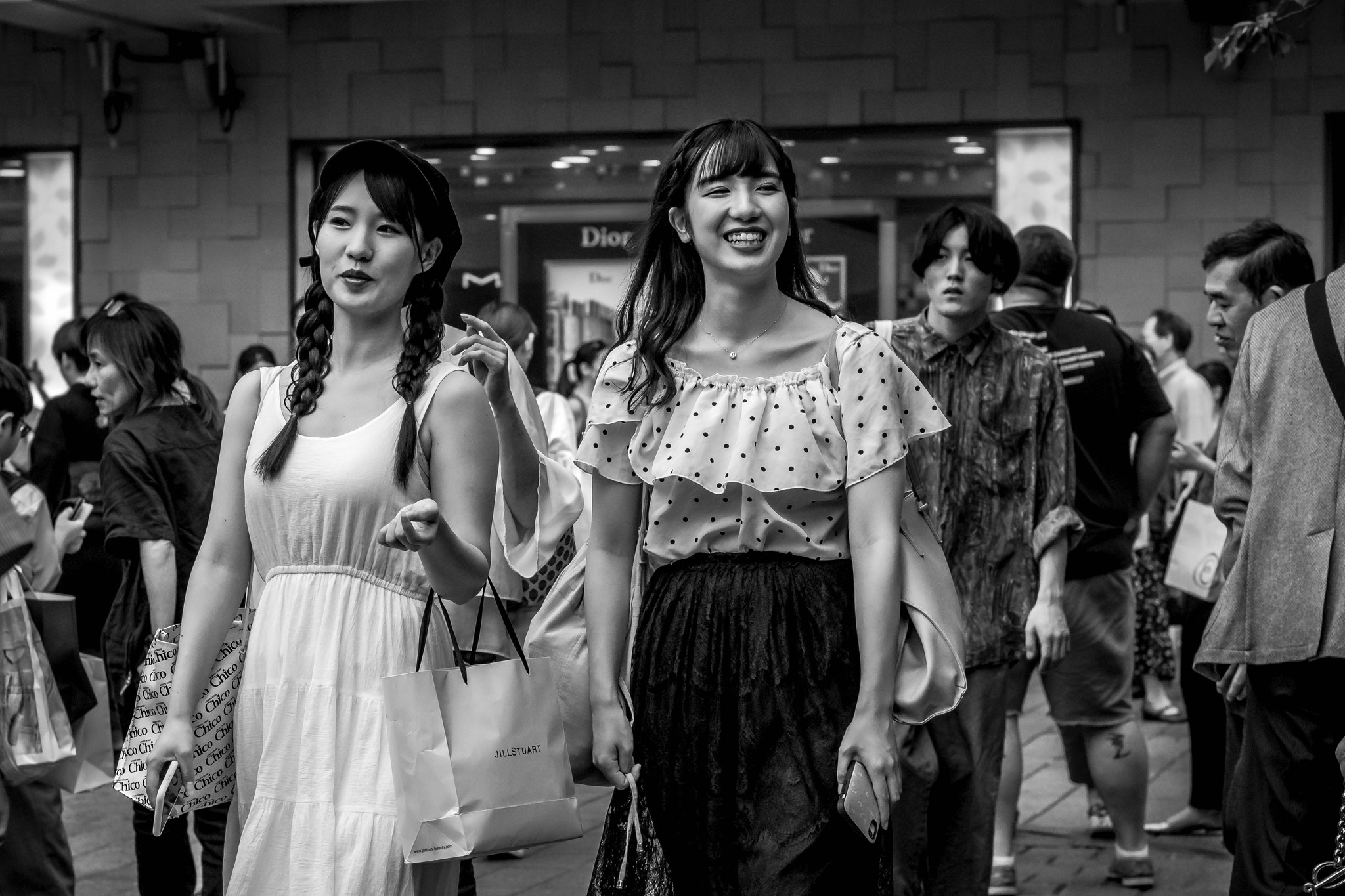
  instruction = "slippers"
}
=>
[1142,701,1187,723]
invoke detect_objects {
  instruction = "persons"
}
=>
[0,224,1345,896]
[572,118,956,895]
[861,202,1085,896]
[142,139,500,896]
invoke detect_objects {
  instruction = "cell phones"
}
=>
[51,495,85,530]
[152,761,182,836]
[838,761,880,844]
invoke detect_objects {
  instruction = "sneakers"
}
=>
[989,864,1016,895]
[1105,854,1157,887]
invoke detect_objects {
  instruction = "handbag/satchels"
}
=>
[830,321,970,724]
[1164,499,1232,601]
[112,554,256,818]
[381,575,583,863]
[525,537,641,787]
[0,567,99,792]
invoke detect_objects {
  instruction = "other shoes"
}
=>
[1145,809,1224,836]
[1087,804,1116,837]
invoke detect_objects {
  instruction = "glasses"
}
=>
[103,299,127,317]
[16,417,34,439]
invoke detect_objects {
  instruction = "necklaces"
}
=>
[697,293,788,359]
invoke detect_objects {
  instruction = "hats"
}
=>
[300,138,464,308]
